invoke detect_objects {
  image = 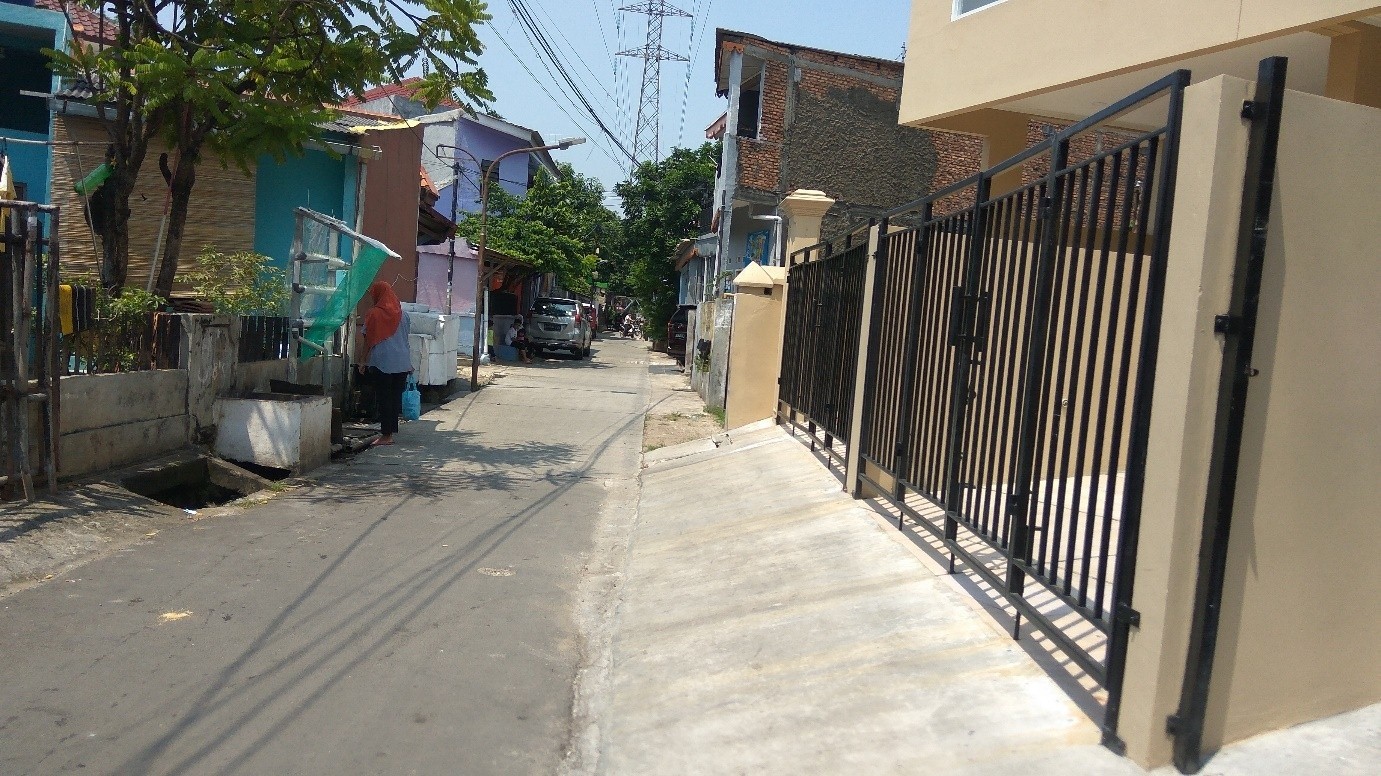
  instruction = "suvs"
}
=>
[524,297,593,359]
[667,302,699,372]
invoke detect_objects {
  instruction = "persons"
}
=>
[504,319,532,364]
[620,312,636,338]
[488,320,497,362]
[358,280,415,447]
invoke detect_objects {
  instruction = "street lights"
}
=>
[469,137,587,393]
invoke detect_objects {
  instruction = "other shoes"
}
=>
[372,439,396,445]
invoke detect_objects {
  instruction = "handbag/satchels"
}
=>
[402,373,423,421]
